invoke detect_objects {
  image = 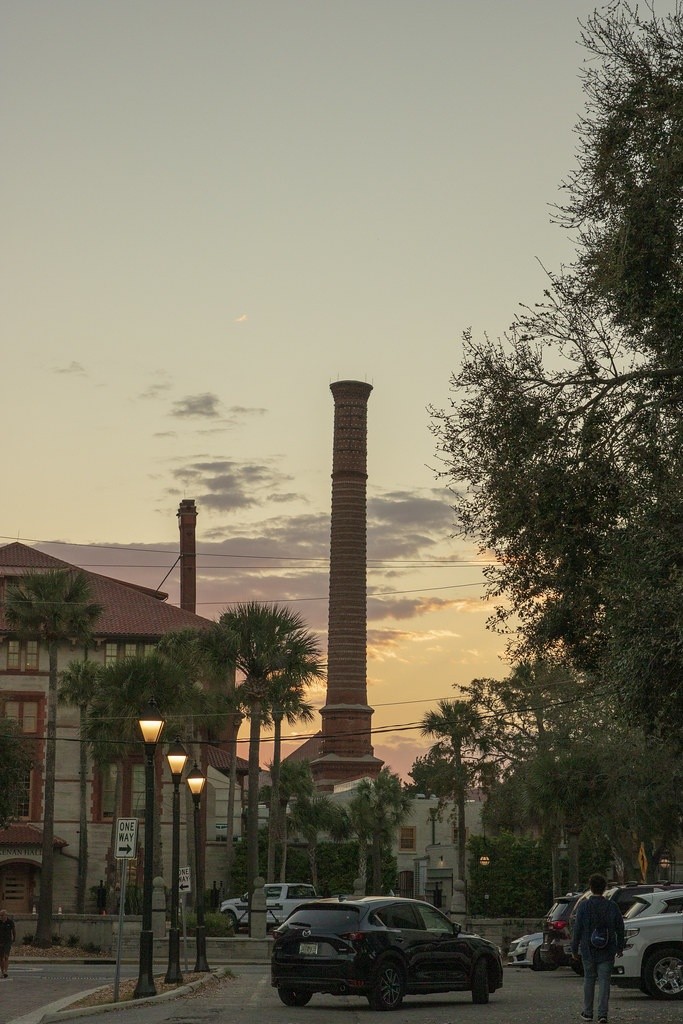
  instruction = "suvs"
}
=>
[269,895,505,1011]
[540,879,683,1001]
[220,880,318,934]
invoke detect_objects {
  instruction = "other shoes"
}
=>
[580,1012,593,1021]
[3,974,8,978]
[597,1016,607,1024]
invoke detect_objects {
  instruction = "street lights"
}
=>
[166,734,189,985]
[129,695,166,1000]
[186,759,206,976]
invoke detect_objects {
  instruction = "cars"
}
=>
[504,931,560,971]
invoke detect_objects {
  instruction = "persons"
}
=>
[0,910,16,978]
[571,874,626,1024]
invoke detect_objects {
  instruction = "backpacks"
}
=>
[585,900,611,951]
